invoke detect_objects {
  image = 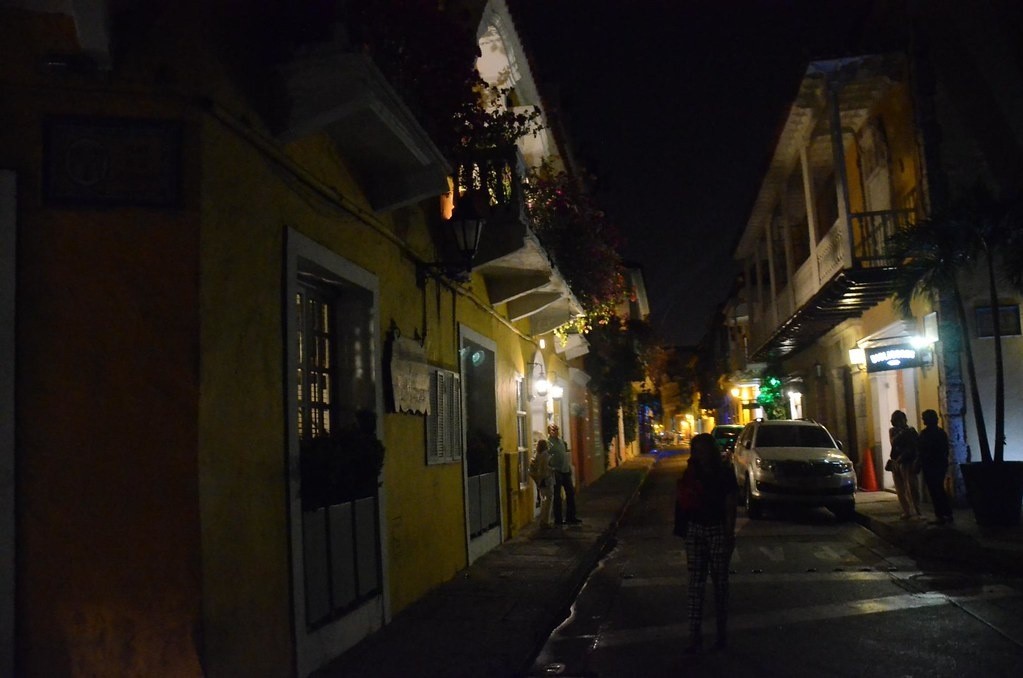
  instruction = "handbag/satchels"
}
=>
[674,518,686,537]
[885,461,893,471]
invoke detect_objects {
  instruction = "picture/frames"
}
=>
[976,303,1023,340]
[40,113,183,209]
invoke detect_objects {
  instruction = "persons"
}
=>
[672,433,740,657]
[919,409,953,526]
[530,439,555,530]
[889,410,926,521]
[547,425,583,528]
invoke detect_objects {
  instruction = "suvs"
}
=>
[731,418,858,518]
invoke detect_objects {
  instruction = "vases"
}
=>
[546,242,584,274]
[567,277,587,302]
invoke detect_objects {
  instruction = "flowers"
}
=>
[454,69,636,338]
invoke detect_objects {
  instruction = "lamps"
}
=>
[813,360,823,379]
[416,186,487,288]
[528,362,547,396]
[547,371,563,401]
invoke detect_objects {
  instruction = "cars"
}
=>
[710,424,746,463]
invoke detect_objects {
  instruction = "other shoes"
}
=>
[901,510,910,519]
[927,516,953,524]
[916,513,927,519]
[710,640,726,652]
[682,645,702,655]
[565,518,582,522]
[553,520,568,525]
[539,523,553,528]
